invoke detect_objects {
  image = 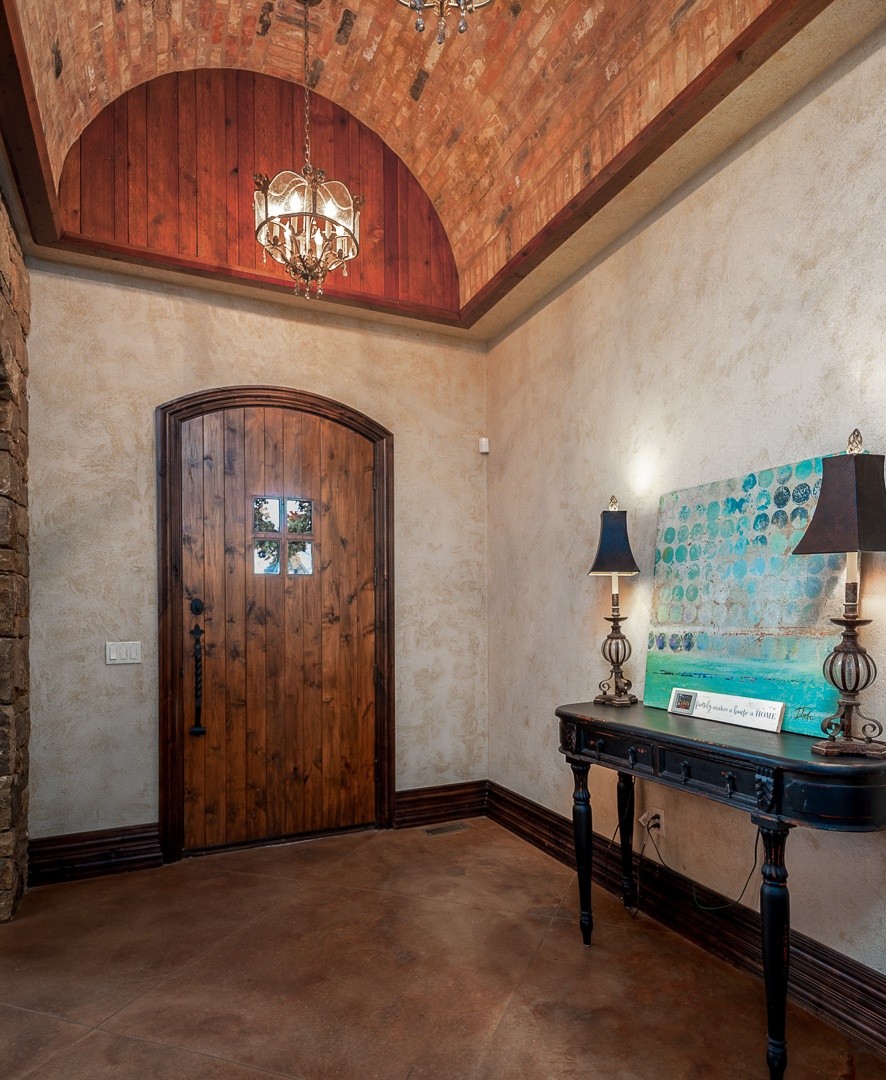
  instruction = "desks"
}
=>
[553,700,885,1080]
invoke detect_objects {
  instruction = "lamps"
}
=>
[587,495,642,707]
[251,0,368,302]
[396,0,492,45]
[793,428,886,759]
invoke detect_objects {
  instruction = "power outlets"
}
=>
[650,807,664,835]
